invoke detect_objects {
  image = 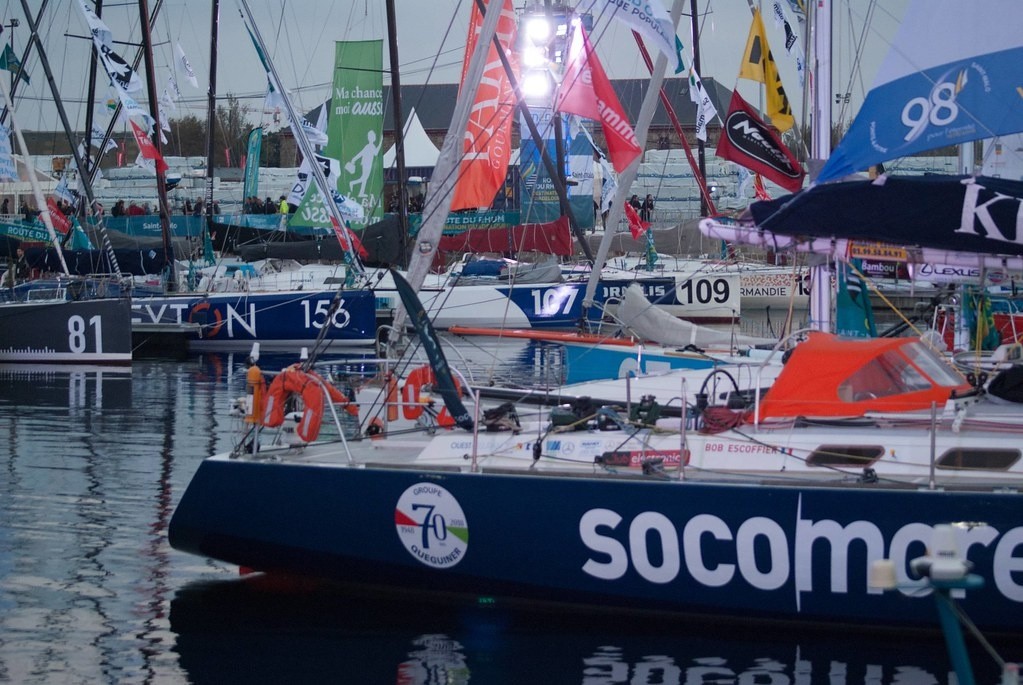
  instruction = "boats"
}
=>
[0,0,1023,628]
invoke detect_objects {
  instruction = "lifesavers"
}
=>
[260,368,326,442]
[402,365,463,427]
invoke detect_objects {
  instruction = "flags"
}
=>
[0,43,30,86]
[37,0,199,253]
[554,0,808,194]
[266,70,368,289]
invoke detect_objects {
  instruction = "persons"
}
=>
[628,195,641,214]
[182,196,221,216]
[194,269,203,289]
[642,193,654,221]
[244,194,289,215]
[0,196,151,220]
[0,247,30,289]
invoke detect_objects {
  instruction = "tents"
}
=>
[382,105,441,169]
